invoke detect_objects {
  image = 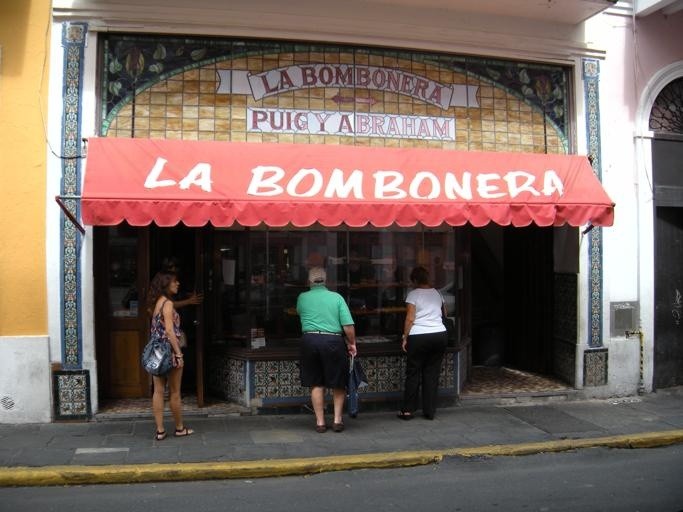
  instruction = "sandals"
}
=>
[397,410,412,420]
[156,427,192,440]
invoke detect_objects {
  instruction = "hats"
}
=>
[309,268,326,284]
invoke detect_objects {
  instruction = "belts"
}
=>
[306,331,342,336]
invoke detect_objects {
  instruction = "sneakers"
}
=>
[316,423,344,433]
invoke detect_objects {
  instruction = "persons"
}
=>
[296,267,358,432]
[166,260,204,309]
[397,267,448,420]
[147,271,204,442]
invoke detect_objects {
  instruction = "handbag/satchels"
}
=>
[442,304,456,349]
[348,354,368,418]
[142,339,175,375]
